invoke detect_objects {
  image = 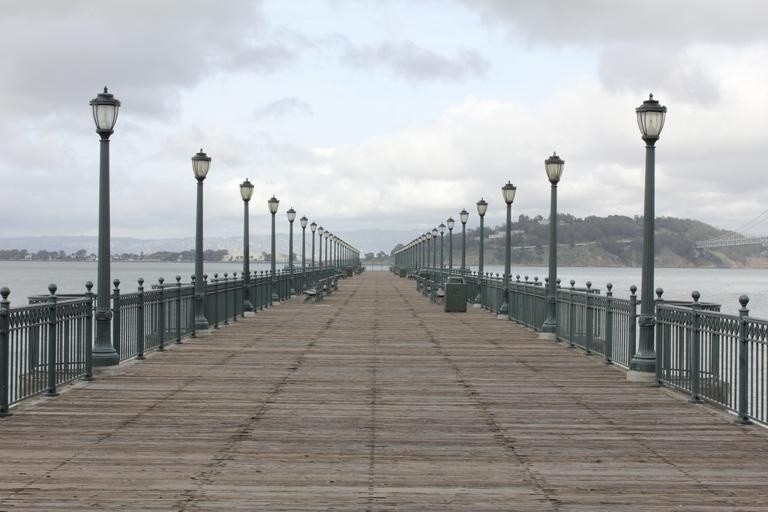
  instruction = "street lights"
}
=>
[189,144,212,330]
[265,193,280,304]
[298,214,360,291]
[238,176,254,313]
[540,149,567,338]
[496,179,519,316]
[457,206,470,272]
[393,221,445,281]
[285,202,297,296]
[82,83,121,368]
[473,194,490,304]
[621,90,672,381]
[445,214,454,275]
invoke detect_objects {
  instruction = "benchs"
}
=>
[389,264,443,306]
[299,265,364,302]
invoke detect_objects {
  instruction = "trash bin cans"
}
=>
[346,266,353,276]
[444,276,467,312]
[399,267,406,277]
